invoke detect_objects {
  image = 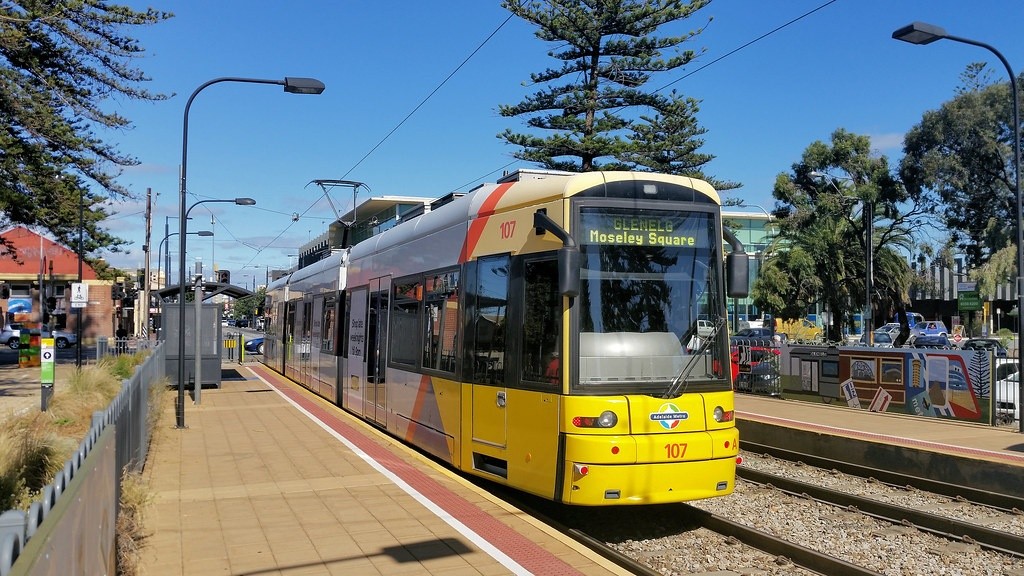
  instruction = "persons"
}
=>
[545,352,559,384]
[115,323,129,354]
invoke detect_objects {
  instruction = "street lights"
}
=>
[255,265,269,288]
[178,75,325,429]
[737,204,775,256]
[164,215,193,287]
[891,22,1024,434]
[169,251,188,286]
[61,179,84,371]
[157,230,214,345]
[243,274,255,330]
[808,170,871,345]
[42,255,53,338]
[237,281,248,291]
[286,254,305,267]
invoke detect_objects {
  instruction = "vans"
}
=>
[737,320,763,335]
[763,319,823,342]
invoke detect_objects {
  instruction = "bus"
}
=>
[263,168,749,507]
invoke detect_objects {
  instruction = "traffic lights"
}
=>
[133,289,138,299]
[111,284,118,300]
[130,289,133,299]
[47,298,56,312]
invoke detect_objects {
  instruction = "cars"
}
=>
[910,335,956,349]
[911,321,948,338]
[698,320,733,337]
[729,327,785,345]
[893,311,925,327]
[221,318,265,330]
[734,362,781,393]
[245,338,263,355]
[688,345,781,382]
[855,332,897,349]
[963,339,1019,422]
[874,323,916,344]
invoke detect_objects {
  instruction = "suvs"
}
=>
[0,324,77,350]
